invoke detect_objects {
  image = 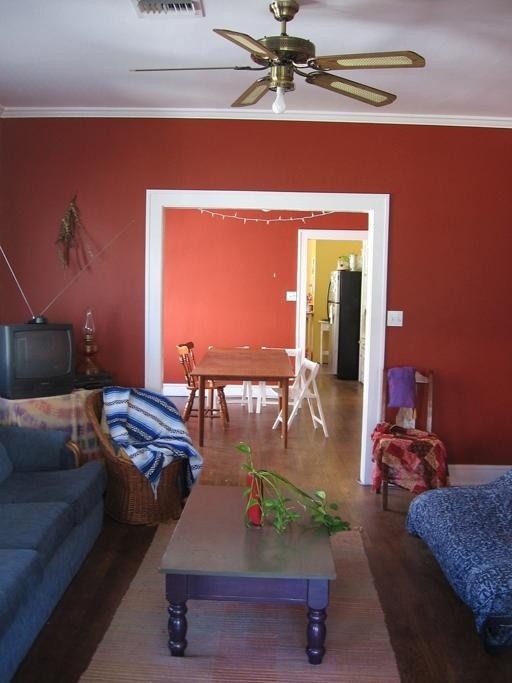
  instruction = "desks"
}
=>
[318,320,331,364]
[190,347,296,449]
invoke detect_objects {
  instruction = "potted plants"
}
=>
[232,442,351,533]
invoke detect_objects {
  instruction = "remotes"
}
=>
[85,382,104,390]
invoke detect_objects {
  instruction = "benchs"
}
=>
[406,468,511,655]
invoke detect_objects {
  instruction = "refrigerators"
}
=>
[326,270,361,380]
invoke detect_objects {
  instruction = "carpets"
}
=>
[78,519,403,683]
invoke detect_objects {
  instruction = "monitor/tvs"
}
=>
[0,323,74,400]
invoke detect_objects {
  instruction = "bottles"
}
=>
[348,252,361,272]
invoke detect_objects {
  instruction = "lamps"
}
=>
[76,306,101,374]
[269,65,295,113]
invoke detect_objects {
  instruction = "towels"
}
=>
[388,366,417,407]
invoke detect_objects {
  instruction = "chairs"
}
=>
[86,388,187,526]
[377,370,446,510]
[256,347,302,415]
[208,345,253,414]
[272,358,329,439]
[176,342,230,423]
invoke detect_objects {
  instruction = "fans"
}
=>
[129,0,425,107]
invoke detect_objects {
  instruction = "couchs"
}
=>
[0,426,107,683]
